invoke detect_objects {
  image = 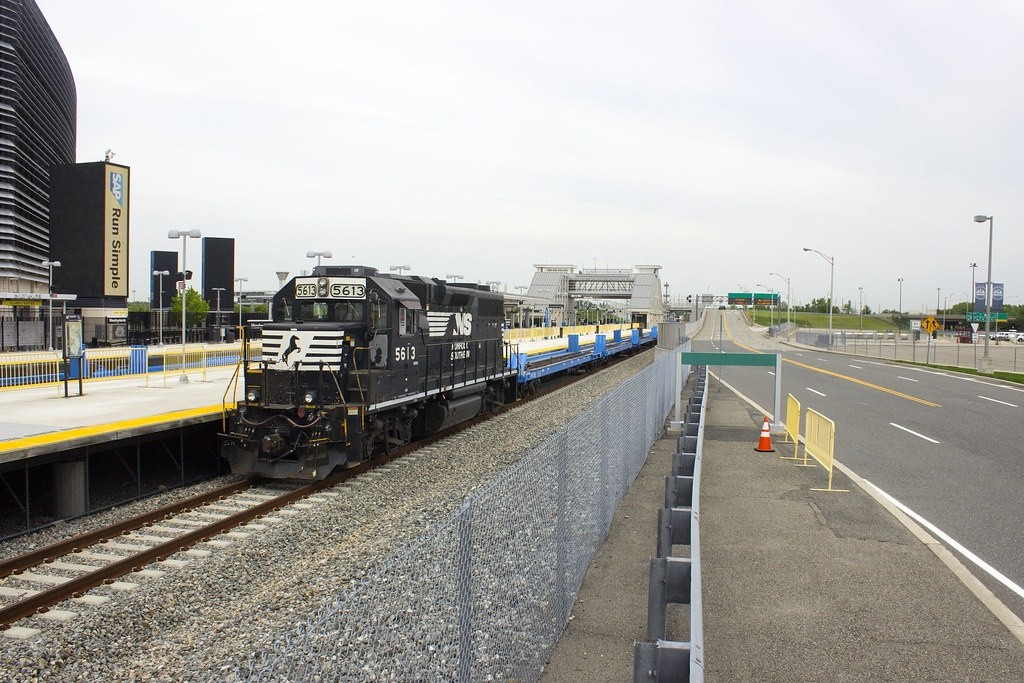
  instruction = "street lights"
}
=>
[390,265,411,275]
[970,262,977,323]
[306,251,332,266]
[975,215,993,373]
[153,270,169,347]
[42,261,62,351]
[770,273,790,336]
[756,284,773,327]
[938,288,941,309]
[446,274,465,283]
[737,284,755,322]
[233,278,248,342]
[515,286,528,295]
[557,292,568,326]
[803,248,834,351]
[584,296,628,326]
[486,281,502,292]
[539,289,550,298]
[944,297,947,338]
[859,287,863,333]
[898,278,904,335]
[571,295,581,326]
[168,229,202,384]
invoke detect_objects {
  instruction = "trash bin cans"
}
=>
[225,327,235,343]
[914,330,920,340]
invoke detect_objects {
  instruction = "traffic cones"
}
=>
[754,416,775,452]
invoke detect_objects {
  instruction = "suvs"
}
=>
[989,332,1024,342]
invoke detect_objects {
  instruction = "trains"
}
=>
[218,265,658,482]
